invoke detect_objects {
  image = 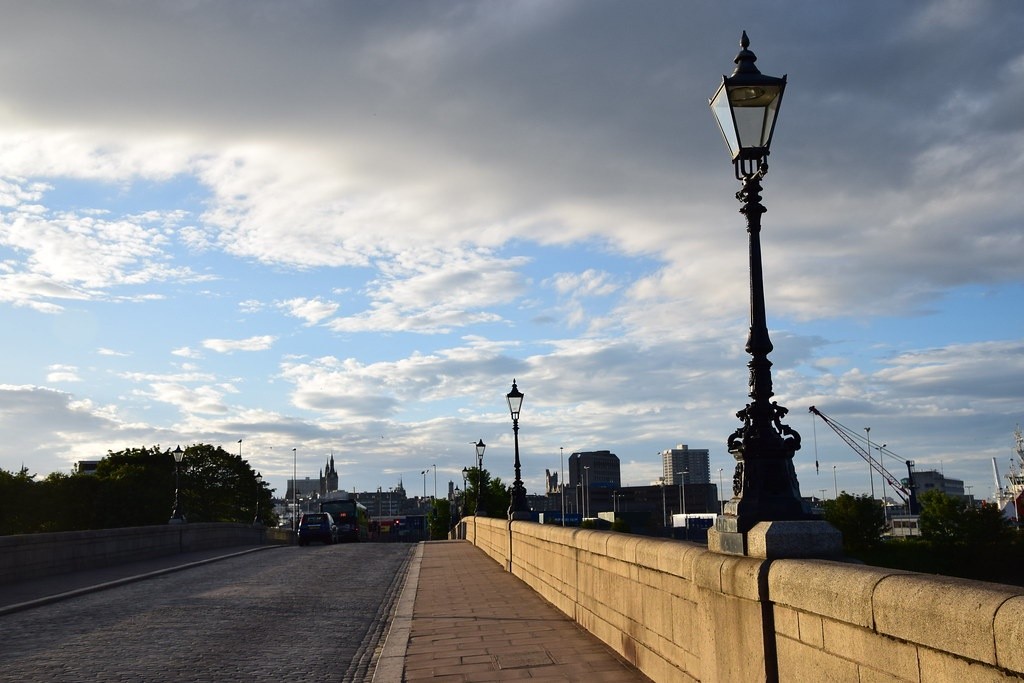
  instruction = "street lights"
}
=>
[474,439,488,517]
[874,443,889,530]
[504,378,532,521]
[420,469,429,540]
[583,465,590,518]
[613,490,618,512]
[679,484,683,515]
[863,427,876,501]
[167,444,186,525]
[575,483,582,519]
[560,446,565,527]
[237,439,243,460]
[377,487,382,525]
[388,487,393,516]
[832,465,838,502]
[469,441,478,507]
[580,474,585,518]
[295,489,301,530]
[292,447,296,533]
[432,464,437,499]
[453,485,462,523]
[707,28,843,557]
[253,471,264,526]
[461,467,471,517]
[676,470,690,513]
[718,468,724,516]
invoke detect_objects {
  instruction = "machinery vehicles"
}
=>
[807,404,919,515]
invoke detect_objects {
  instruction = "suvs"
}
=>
[298,512,338,546]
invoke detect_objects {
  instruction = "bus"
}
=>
[321,498,370,543]
